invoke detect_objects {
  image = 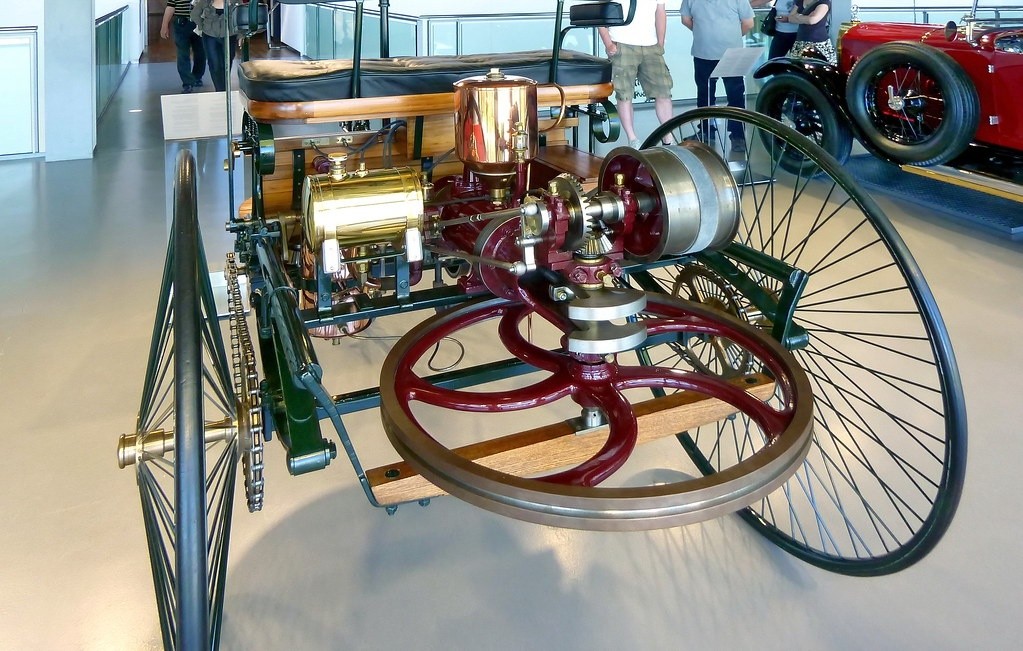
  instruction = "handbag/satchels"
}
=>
[761,0,777,36]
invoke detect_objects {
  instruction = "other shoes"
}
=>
[194,78,203,87]
[628,139,641,150]
[181,85,192,94]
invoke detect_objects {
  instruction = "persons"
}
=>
[750,0,837,77]
[678,0,755,153]
[596,0,675,151]
[189,0,244,92]
[161,0,206,95]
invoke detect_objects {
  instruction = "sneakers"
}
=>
[683,129,715,147]
[731,138,745,152]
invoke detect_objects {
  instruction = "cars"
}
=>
[752,0,1023,206]
